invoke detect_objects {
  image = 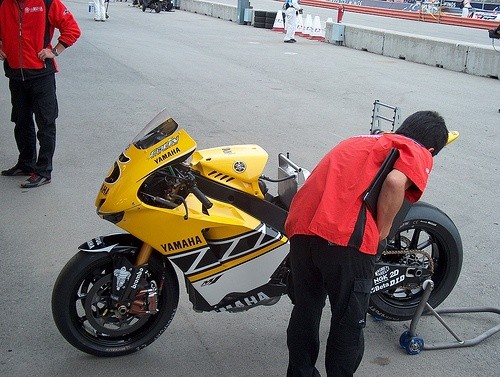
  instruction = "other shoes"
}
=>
[290,38,296,42]
[284,40,295,43]
[94,18,100,21]
[101,20,105,22]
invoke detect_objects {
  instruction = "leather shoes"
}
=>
[2,163,34,176]
[21,174,51,188]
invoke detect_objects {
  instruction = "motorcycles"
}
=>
[51,99,500,358]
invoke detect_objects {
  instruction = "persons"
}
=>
[284,110,449,377]
[94,0,109,20]
[0,0,81,188]
[282,0,303,42]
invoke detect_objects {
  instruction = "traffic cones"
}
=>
[295,13,303,35]
[270,10,284,32]
[318,18,332,42]
[299,15,312,37]
[284,15,287,34]
[308,16,323,41]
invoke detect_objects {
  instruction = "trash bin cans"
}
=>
[174,0,180,9]
[236,0,249,24]
